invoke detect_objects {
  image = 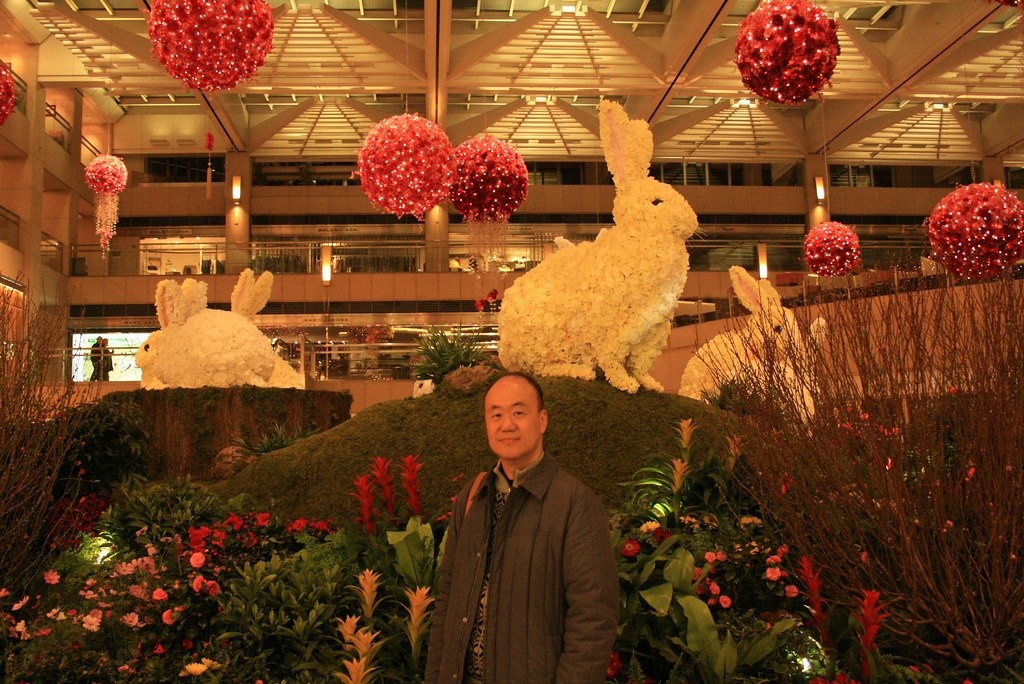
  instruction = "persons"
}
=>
[424,372,619,682]
[90,336,114,381]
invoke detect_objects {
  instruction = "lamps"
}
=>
[814,175,825,206]
[755,240,769,280]
[321,246,331,286]
[232,175,241,206]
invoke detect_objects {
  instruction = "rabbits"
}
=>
[499,99,703,393]
[135,268,305,389]
[676,264,825,434]
